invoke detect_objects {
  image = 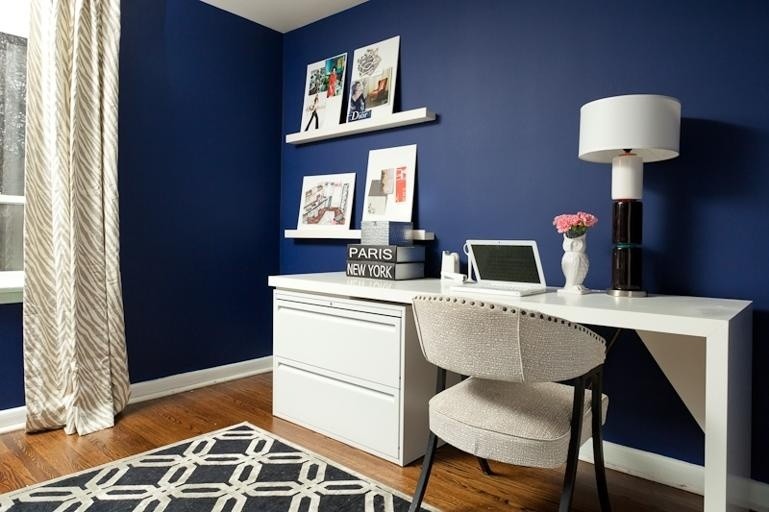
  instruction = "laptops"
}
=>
[452,239,547,297]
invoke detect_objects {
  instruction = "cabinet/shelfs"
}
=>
[272,288,462,466]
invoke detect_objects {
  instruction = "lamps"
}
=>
[578,94,682,299]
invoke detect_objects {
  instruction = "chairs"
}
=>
[404,291,614,512]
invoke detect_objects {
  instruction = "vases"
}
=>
[558,234,591,296]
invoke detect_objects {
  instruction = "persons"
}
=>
[327,66,338,98]
[304,94,320,130]
[351,80,368,119]
[314,69,322,88]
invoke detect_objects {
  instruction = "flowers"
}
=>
[554,210,600,237]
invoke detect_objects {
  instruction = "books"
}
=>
[345,219,427,280]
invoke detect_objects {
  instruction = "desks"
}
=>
[406,276,753,512]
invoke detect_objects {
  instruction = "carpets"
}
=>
[1,421,442,512]
